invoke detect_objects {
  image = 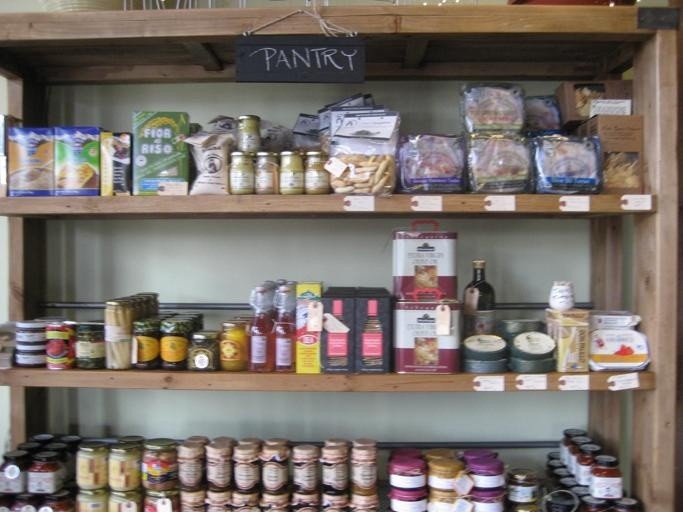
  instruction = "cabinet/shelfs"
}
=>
[0,6,681,512]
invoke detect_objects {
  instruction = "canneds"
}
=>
[1,292,253,373]
[139,436,380,510]
[387,447,507,511]
[540,429,641,512]
[506,468,540,505]
[0,428,145,512]
[228,114,330,194]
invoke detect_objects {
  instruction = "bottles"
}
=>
[328,301,349,366]
[412,313,439,367]
[413,243,438,294]
[357,300,383,367]
[508,431,647,510]
[547,280,574,311]
[248,278,297,374]
[461,257,495,312]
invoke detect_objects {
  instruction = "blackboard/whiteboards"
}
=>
[234,35,365,83]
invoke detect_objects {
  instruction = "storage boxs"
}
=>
[577,115,644,195]
[556,79,634,126]
